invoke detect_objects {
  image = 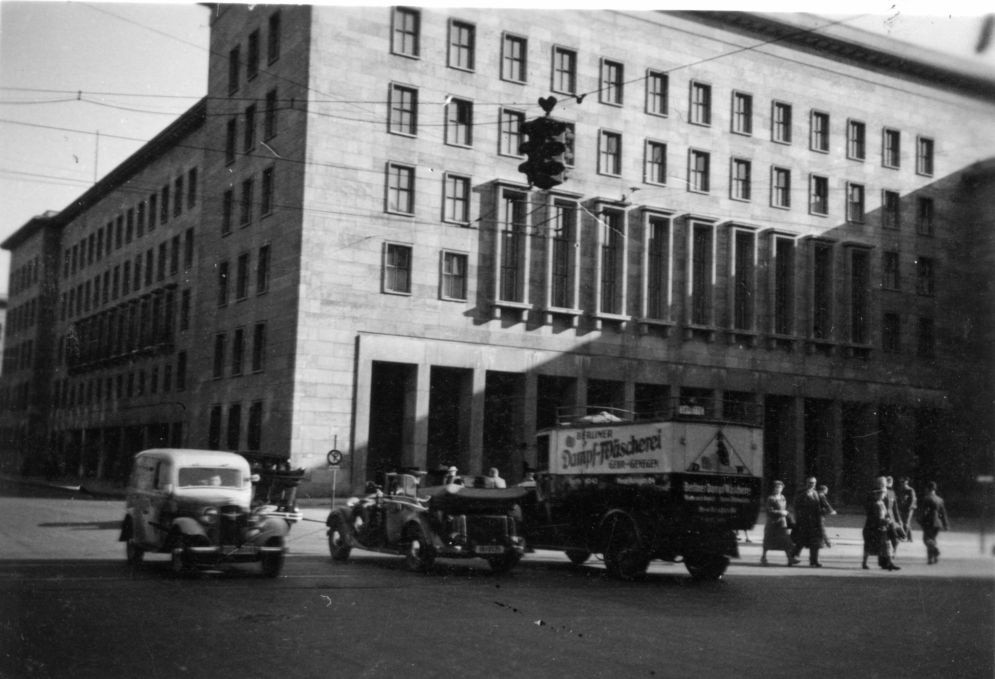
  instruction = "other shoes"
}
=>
[932,547,940,563]
[790,557,800,564]
[888,564,901,571]
[760,556,767,564]
[809,561,823,567]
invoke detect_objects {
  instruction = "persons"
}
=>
[443,466,464,488]
[862,476,949,571]
[488,468,506,488]
[208,471,223,486]
[518,468,536,487]
[396,467,428,499]
[736,529,752,543]
[760,477,836,567]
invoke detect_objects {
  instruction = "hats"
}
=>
[448,465,458,472]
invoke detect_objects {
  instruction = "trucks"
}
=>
[510,403,766,587]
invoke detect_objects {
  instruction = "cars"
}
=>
[327,470,534,577]
[119,448,292,580]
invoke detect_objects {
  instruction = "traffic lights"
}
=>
[537,118,567,185]
[518,118,539,183]
